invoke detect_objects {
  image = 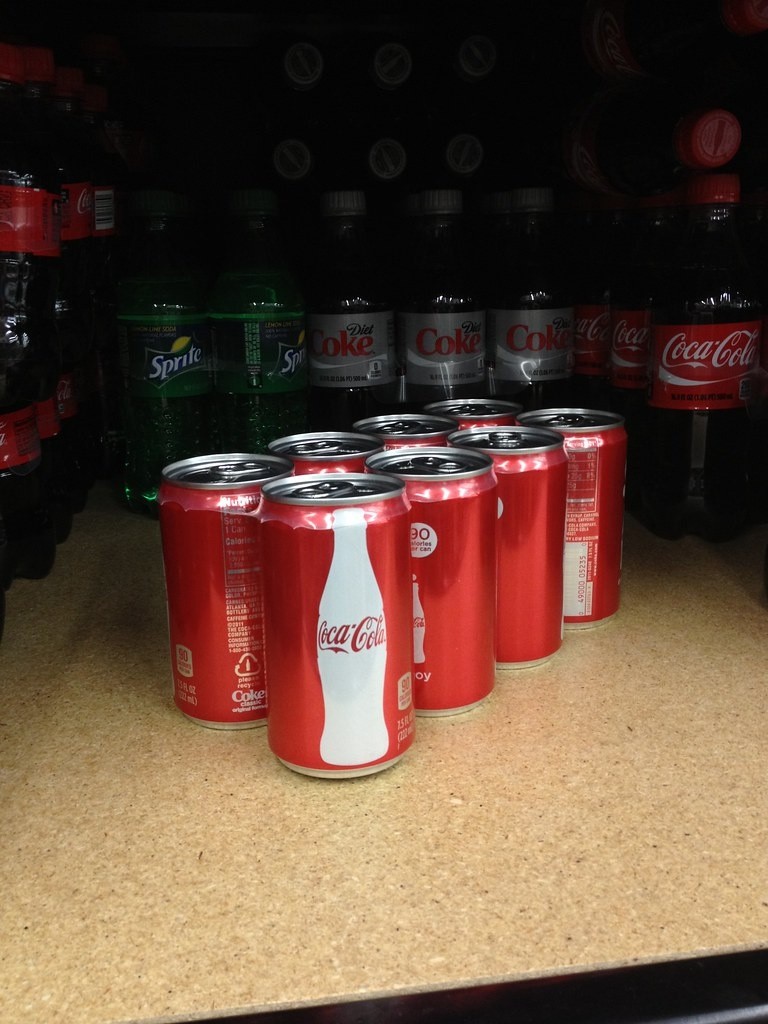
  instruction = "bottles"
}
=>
[0,0,768,638]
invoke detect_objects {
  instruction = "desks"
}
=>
[0,475,768,1024]
